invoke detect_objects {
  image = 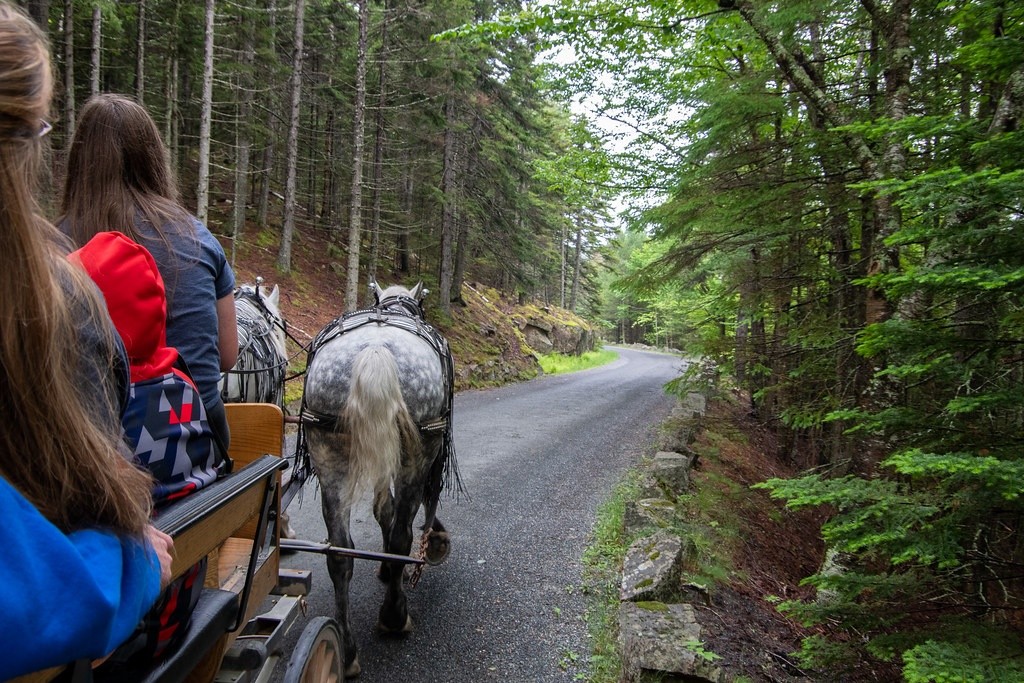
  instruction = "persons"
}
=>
[51,92,238,481]
[1,1,155,571]
[65,230,217,653]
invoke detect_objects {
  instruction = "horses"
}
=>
[217,283,296,555]
[302,277,455,679]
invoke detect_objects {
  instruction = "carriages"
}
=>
[4,269,472,683]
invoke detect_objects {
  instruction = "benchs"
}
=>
[210,403,282,606]
